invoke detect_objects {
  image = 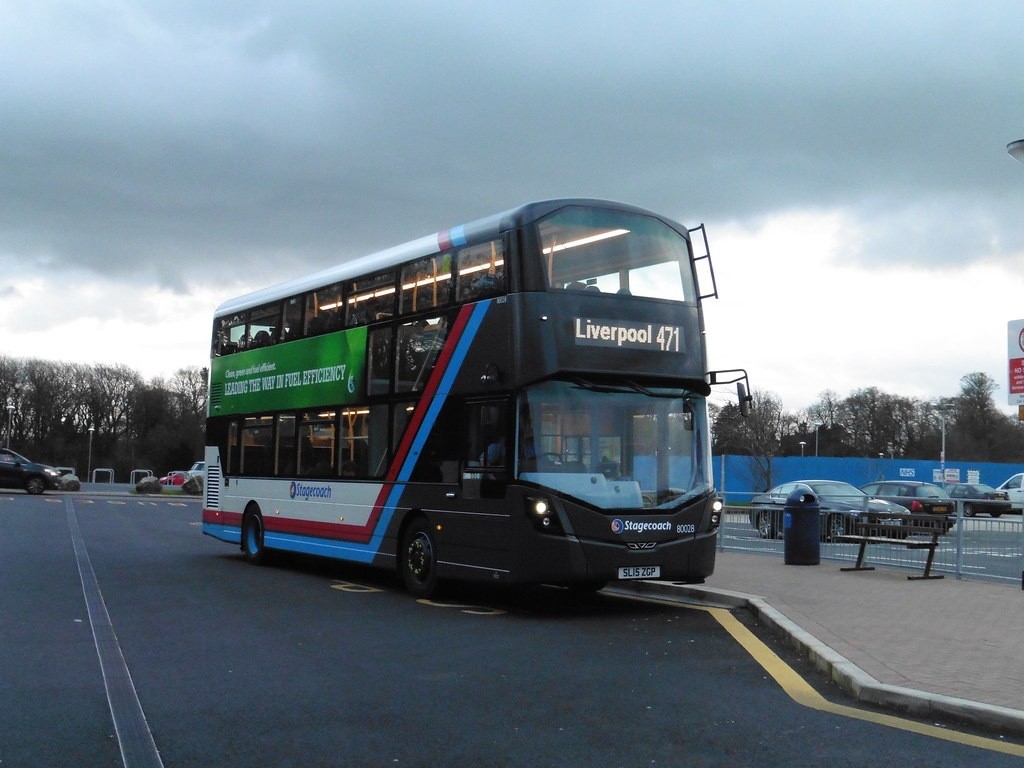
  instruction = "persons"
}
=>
[480,428,507,480]
[525,437,542,457]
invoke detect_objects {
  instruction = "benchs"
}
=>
[832,511,948,582]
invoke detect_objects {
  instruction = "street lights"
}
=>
[88,427,95,483]
[6,401,15,449]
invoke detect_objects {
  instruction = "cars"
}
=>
[187,462,205,478]
[943,483,1012,517]
[858,480,957,534]
[749,480,913,542]
[996,473,1024,509]
[159,473,189,486]
[0,448,64,494]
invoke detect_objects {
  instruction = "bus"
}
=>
[201,197,753,596]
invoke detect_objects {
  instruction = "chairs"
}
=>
[238,311,340,352]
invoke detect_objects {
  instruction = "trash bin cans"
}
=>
[783,485,820,566]
